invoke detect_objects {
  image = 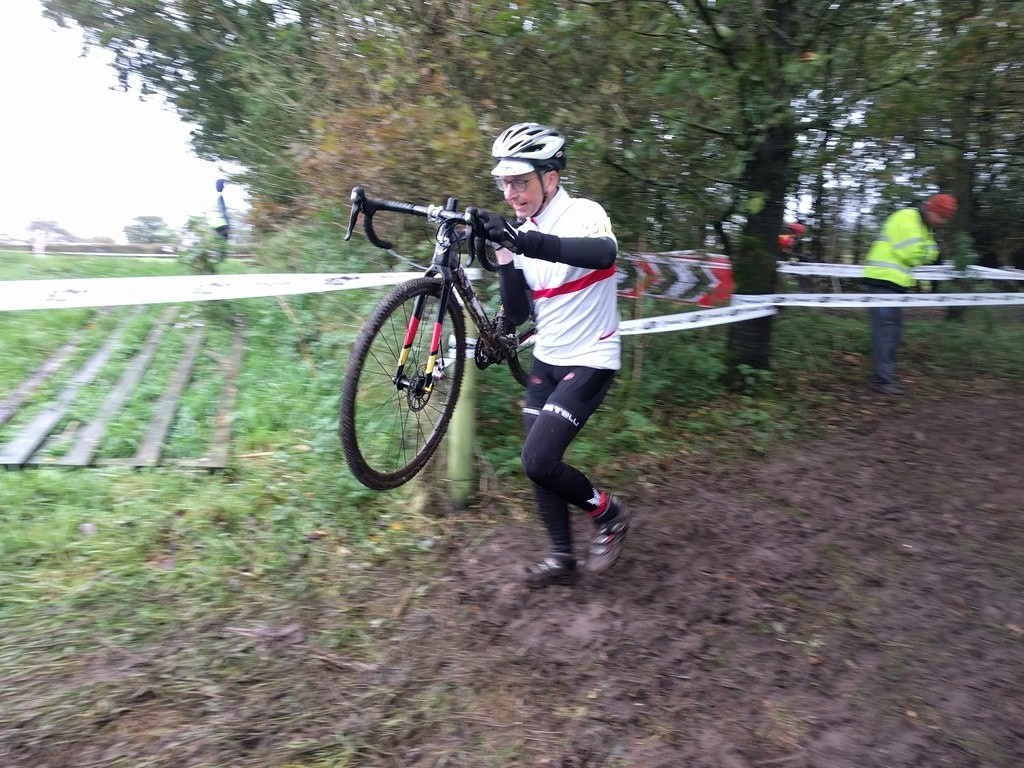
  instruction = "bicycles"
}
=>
[333,183,534,493]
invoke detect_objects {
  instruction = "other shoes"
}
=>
[869,374,906,395]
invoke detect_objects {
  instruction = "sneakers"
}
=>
[585,493,632,573]
[523,552,576,587]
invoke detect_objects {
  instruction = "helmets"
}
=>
[790,224,806,234]
[926,194,960,217]
[778,235,794,247]
[491,122,566,176]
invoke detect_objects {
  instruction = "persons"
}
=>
[863,194,959,396]
[778,222,807,262]
[474,122,631,587]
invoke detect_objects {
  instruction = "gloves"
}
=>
[478,210,518,255]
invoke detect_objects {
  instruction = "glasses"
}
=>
[496,176,538,192]
